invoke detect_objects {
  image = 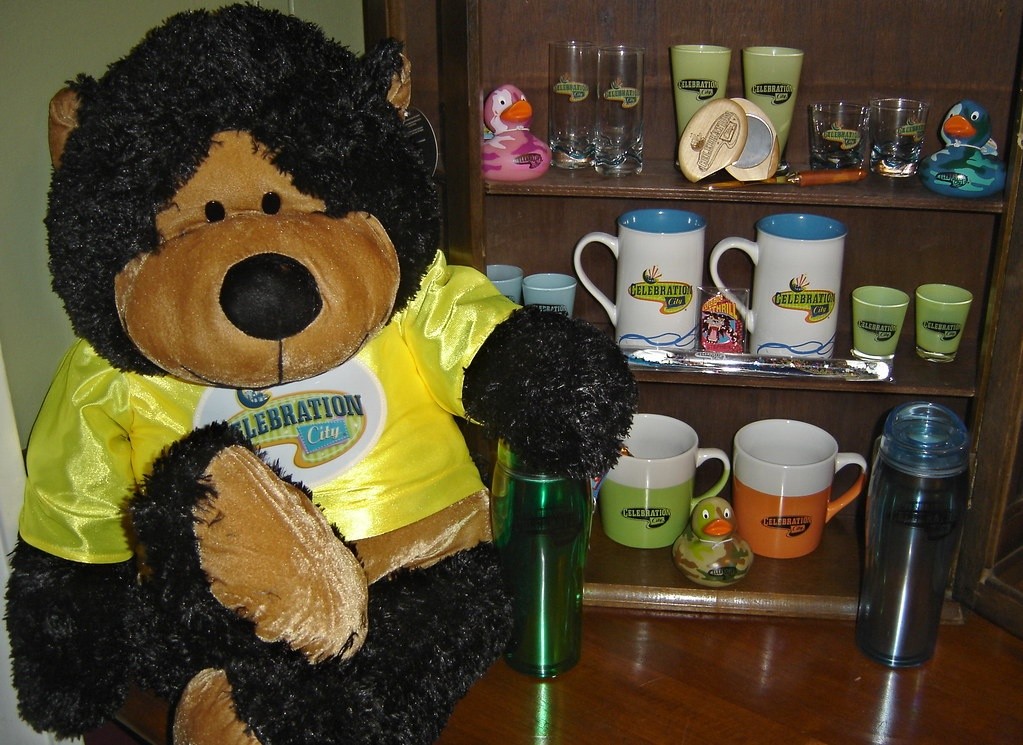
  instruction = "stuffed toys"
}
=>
[3,3,639,745]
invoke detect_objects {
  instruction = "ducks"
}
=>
[481,84,551,179]
[918,100,1006,198]
[672,497,753,587]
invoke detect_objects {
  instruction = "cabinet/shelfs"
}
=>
[356,0,1023,620]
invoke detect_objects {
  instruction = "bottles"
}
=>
[855,402,973,668]
[490,438,595,679]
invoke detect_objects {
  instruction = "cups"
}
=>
[547,39,644,178]
[809,102,870,168]
[732,418,868,559]
[669,42,803,163]
[872,98,932,178]
[913,283,973,363]
[708,213,850,359]
[599,412,731,549]
[487,264,524,305]
[572,208,707,347]
[521,272,577,317]
[851,286,910,361]
